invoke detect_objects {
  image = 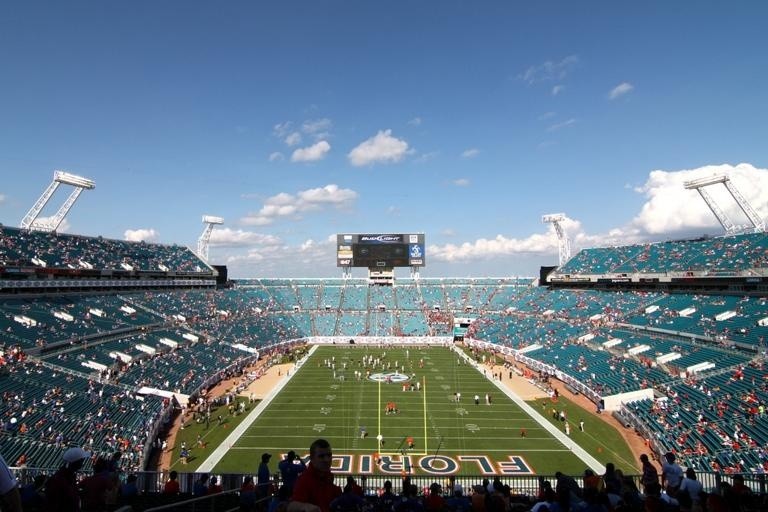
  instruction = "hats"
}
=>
[61,446,92,463]
[262,453,272,460]
[342,477,555,497]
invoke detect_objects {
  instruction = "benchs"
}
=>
[612,405,767,480]
[254,279,542,346]
[514,231,767,405]
[2,404,168,484]
[1,222,266,404]
